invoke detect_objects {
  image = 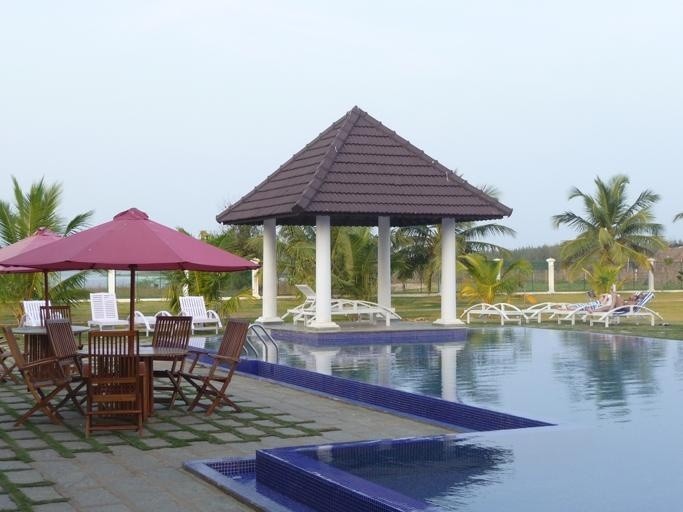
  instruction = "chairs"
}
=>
[459,290,664,327]
[280,282,402,326]
[1,291,251,440]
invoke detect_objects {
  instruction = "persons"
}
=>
[558,294,611,313]
[586,292,639,314]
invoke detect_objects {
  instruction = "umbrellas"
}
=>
[0,226,87,325]
[0,205,263,352]
